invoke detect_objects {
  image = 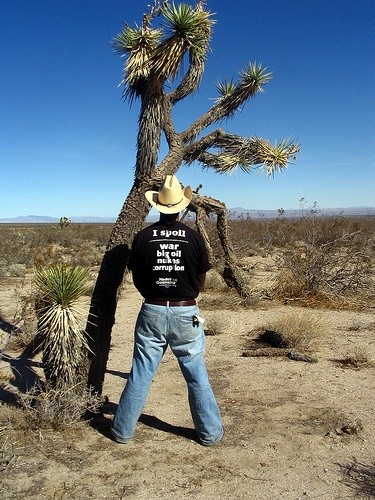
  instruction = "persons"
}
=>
[105,175,226,446]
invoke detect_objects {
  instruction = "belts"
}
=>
[145,299,195,307]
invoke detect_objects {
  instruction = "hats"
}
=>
[145,174,194,214]
